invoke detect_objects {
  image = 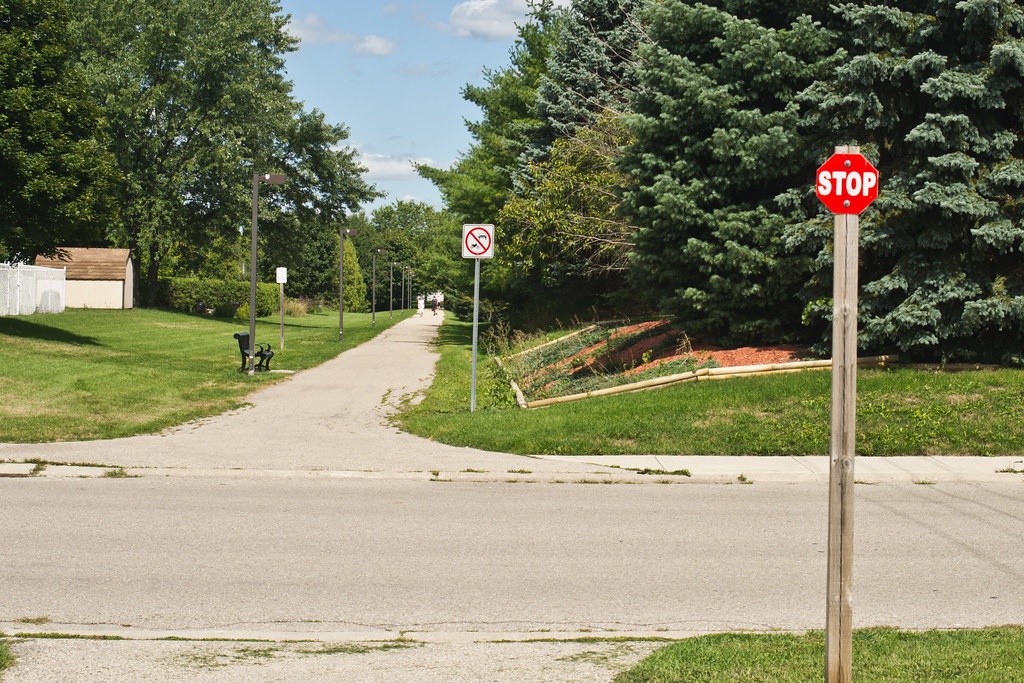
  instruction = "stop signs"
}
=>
[814,153,880,216]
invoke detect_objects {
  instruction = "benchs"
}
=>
[233,330,274,371]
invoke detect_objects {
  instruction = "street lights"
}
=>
[337,227,359,344]
[388,262,401,319]
[370,248,385,330]
[402,265,410,313]
[407,271,414,310]
[247,171,287,377]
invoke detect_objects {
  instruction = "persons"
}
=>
[418,298,425,316]
[431,295,438,316]
